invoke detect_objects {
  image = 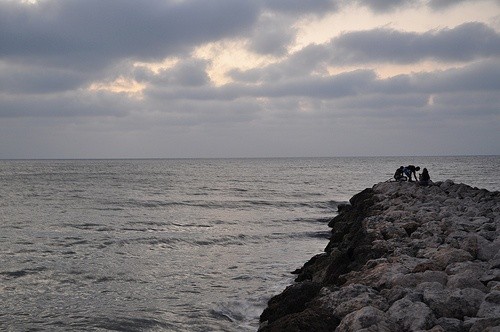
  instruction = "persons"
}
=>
[419,167,430,182]
[404,164,420,182]
[394,165,407,182]
